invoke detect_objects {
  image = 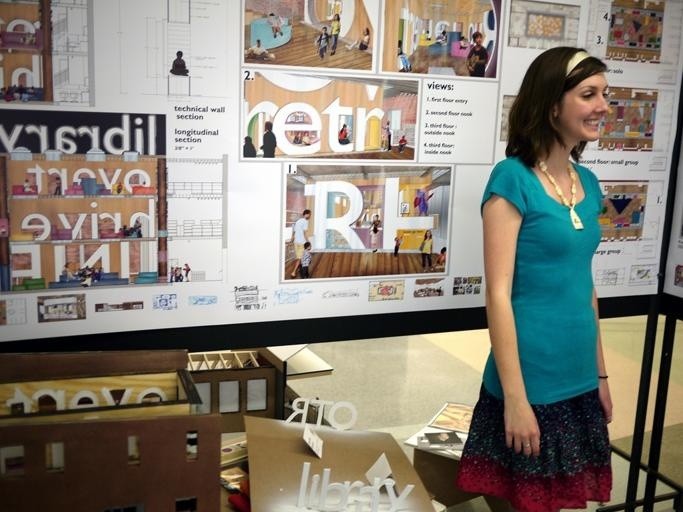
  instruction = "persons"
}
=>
[455,45,613,512]
[0,0,489,289]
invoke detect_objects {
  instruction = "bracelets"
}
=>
[599,373,610,380]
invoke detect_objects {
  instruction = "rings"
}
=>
[522,442,530,448]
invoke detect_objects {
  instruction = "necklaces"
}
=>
[535,159,586,231]
